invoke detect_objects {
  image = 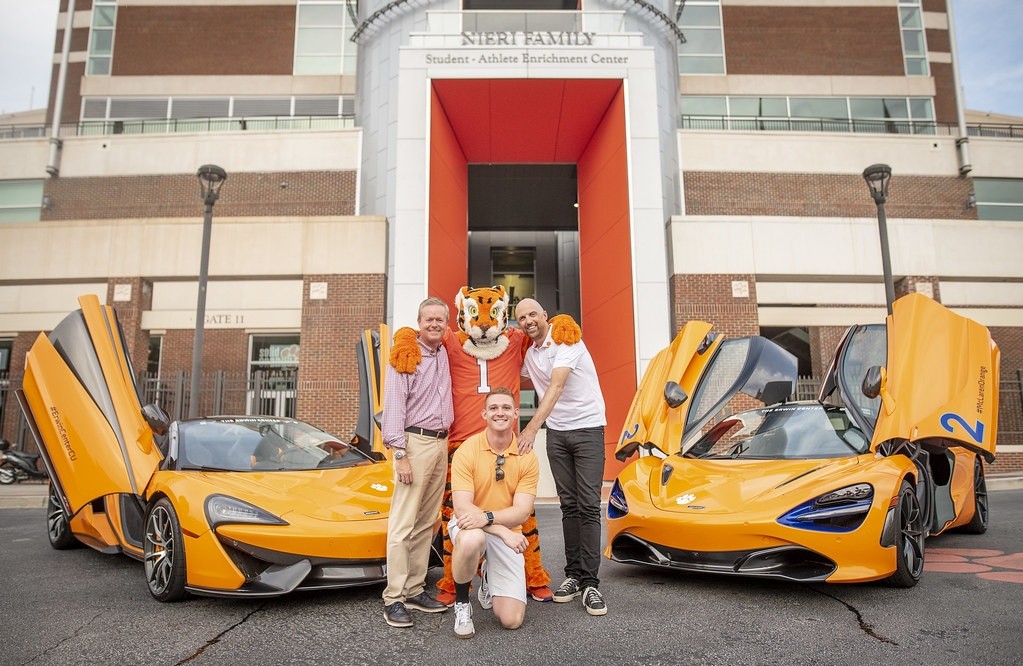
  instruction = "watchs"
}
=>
[394,450,407,460]
[483,510,494,526]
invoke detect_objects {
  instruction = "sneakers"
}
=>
[435,590,457,607]
[403,592,448,613]
[478,559,493,610]
[553,577,582,603]
[582,586,607,615]
[527,586,554,602]
[383,601,413,628]
[453,601,475,639]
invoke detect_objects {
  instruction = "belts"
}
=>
[404,426,448,439]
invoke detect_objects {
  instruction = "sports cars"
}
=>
[604,292,1001,588]
[14,294,444,603]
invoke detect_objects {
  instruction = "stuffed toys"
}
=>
[390,284,582,609]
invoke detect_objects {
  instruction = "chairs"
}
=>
[186,432,213,466]
[229,437,260,466]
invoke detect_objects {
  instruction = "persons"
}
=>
[515,298,607,615]
[448,387,539,639]
[380,298,455,628]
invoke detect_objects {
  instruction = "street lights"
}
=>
[188,164,228,419]
[862,164,896,315]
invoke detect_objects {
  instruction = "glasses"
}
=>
[495,454,505,481]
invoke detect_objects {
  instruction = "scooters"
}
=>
[0,439,50,485]
